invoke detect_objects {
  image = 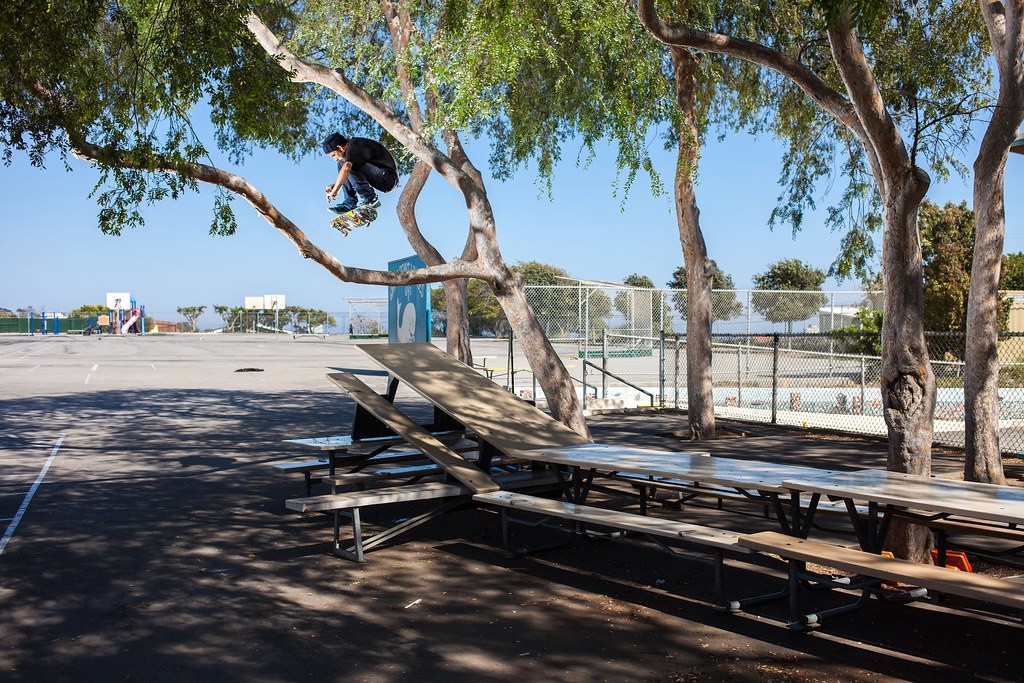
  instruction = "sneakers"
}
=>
[360,196,381,208]
[328,201,358,214]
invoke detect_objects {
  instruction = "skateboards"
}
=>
[329,205,378,237]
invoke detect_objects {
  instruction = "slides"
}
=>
[119,315,138,335]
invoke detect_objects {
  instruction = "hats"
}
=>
[322,132,347,154]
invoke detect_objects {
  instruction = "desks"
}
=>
[281,430,480,497]
[739,467,1024,629]
[472,443,851,613]
[327,343,594,493]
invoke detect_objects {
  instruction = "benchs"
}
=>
[595,468,1024,544]
[321,457,533,491]
[472,490,767,557]
[271,448,428,495]
[327,373,500,494]
[285,467,574,565]
[738,530,1024,611]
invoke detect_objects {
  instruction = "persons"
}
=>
[323,133,399,215]
